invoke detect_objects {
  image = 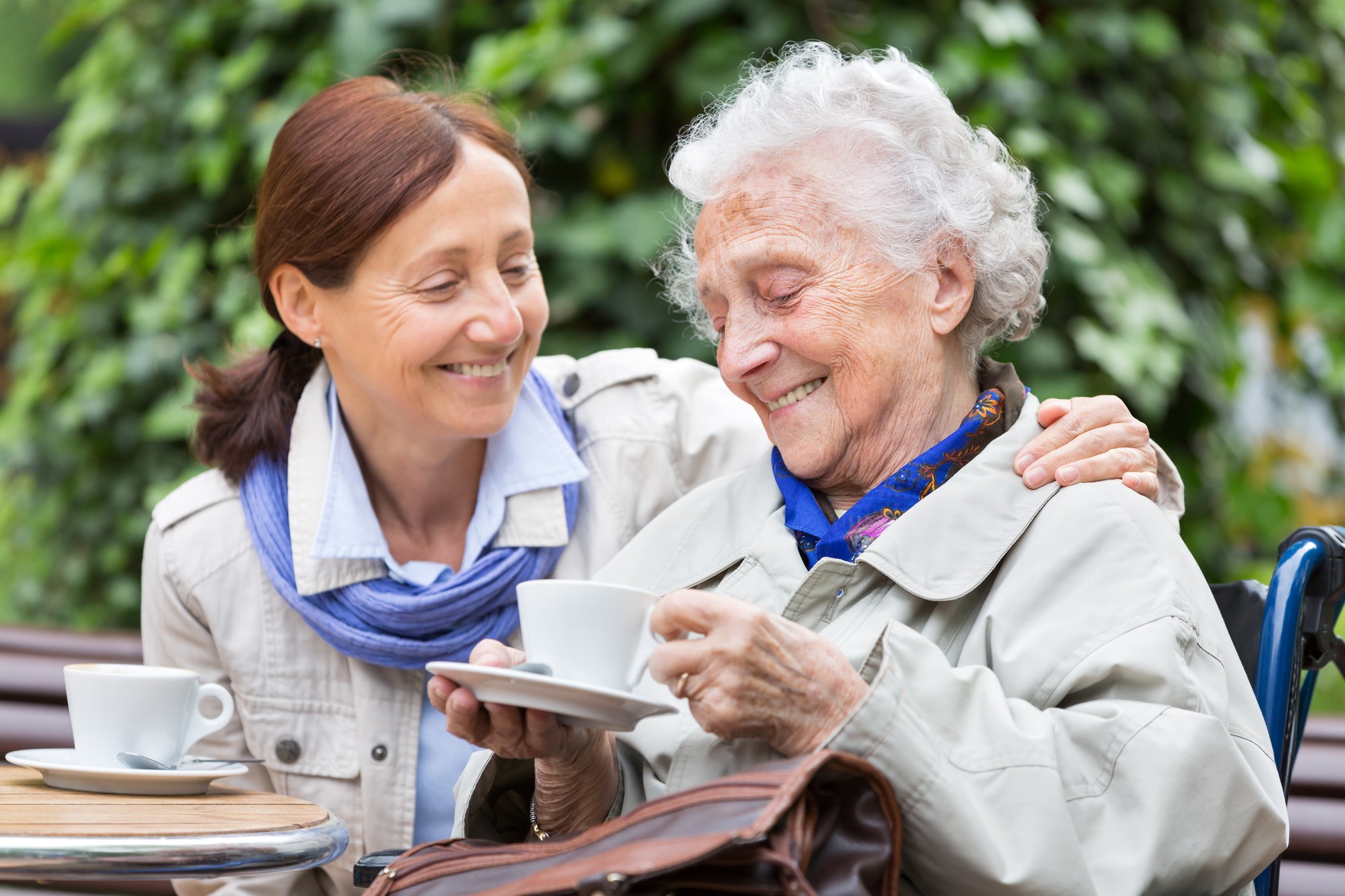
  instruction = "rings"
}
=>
[676,672,690,700]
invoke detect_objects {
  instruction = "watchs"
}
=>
[530,787,551,844]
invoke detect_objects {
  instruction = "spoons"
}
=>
[516,663,552,676]
[117,752,264,770]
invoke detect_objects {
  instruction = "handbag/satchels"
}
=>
[364,753,902,896]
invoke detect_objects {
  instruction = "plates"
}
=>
[425,661,679,732]
[5,748,249,795]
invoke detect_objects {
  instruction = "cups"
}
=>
[515,579,663,692]
[62,663,235,770]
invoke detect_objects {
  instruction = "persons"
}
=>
[424,35,1290,894]
[135,77,1188,893]
